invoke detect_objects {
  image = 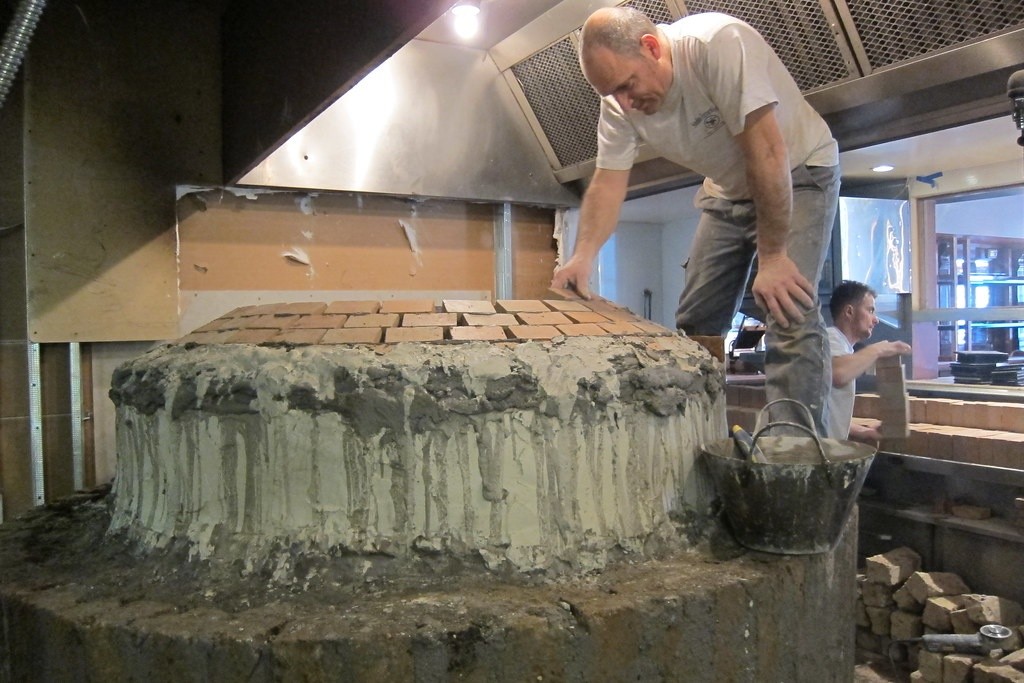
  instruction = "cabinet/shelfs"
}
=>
[935,232,1024,370]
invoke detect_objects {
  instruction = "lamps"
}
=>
[450,1,483,42]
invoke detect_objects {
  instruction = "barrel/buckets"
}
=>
[701,398,876,554]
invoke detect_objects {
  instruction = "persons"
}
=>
[824,280,913,444]
[551,5,841,439]
